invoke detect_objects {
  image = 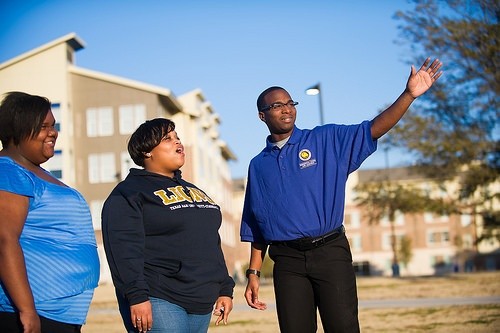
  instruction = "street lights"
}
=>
[382,138,402,277]
[304,83,324,125]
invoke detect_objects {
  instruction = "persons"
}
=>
[102,118,235,333]
[241,58,444,333]
[0,91,100,333]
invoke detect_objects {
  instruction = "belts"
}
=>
[280,227,347,250]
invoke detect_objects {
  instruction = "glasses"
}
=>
[261,101,300,111]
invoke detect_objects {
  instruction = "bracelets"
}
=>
[246,269,261,277]
